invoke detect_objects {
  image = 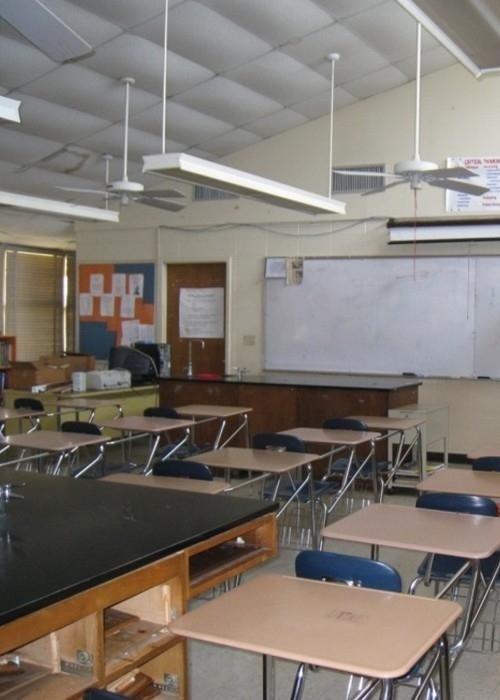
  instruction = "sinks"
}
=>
[220,375,234,377]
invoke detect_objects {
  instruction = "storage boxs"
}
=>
[6,352,96,391]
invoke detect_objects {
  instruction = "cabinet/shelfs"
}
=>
[388,403,451,494]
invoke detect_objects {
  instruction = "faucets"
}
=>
[187,339,205,377]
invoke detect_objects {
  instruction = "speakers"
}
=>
[72,372,85,392]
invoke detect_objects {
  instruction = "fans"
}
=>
[332,23,491,197]
[55,77,186,212]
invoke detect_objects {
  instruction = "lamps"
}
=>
[391,0,500,81]
[141,0,348,216]
[0,155,120,223]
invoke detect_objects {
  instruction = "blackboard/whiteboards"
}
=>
[262,254,500,381]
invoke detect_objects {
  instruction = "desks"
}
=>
[3,385,169,440]
[275,428,380,494]
[415,469,500,498]
[319,503,500,654]
[183,446,319,505]
[344,415,426,468]
[1,466,277,700]
[95,472,231,495]
[164,574,463,700]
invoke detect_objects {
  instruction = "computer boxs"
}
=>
[136,343,171,378]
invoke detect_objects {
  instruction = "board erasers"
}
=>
[478,376,490,379]
[403,372,414,376]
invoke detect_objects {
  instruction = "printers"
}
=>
[86,368,131,390]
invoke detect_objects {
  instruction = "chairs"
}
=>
[322,417,393,480]
[1,399,252,477]
[472,456,500,471]
[252,433,340,537]
[295,551,400,594]
[408,493,500,672]
